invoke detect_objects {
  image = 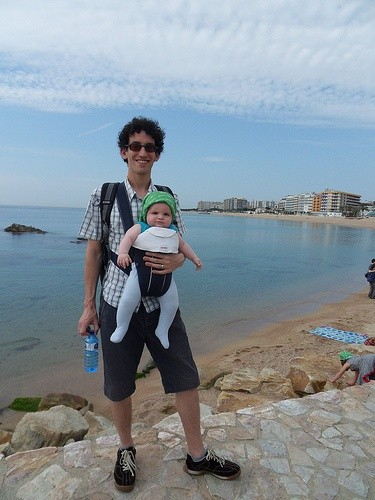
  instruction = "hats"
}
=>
[338,350,351,361]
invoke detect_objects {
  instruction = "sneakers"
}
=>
[186,451,242,480]
[113,445,137,492]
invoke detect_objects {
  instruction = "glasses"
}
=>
[124,141,157,153]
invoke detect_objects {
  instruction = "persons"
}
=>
[78,117,241,493]
[327,351,375,386]
[365,259,375,299]
[110,191,201,349]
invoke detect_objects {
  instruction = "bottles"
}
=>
[83,330,99,373]
